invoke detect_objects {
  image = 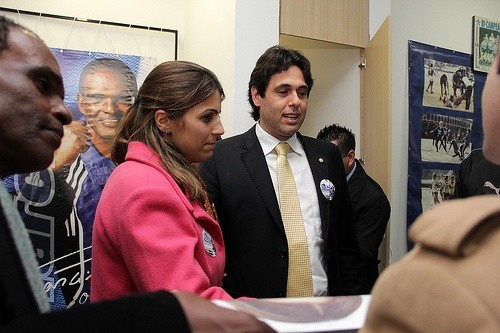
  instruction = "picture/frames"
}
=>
[472,15,500,74]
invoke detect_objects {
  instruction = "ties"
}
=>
[274,143,313,298]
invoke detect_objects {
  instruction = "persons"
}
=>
[317,123,390,298]
[90,61,260,299]
[0,16,270,333]
[49,57,149,296]
[199,45,361,300]
[357,40,500,333]
[452,147,500,200]
[426,33,500,204]
